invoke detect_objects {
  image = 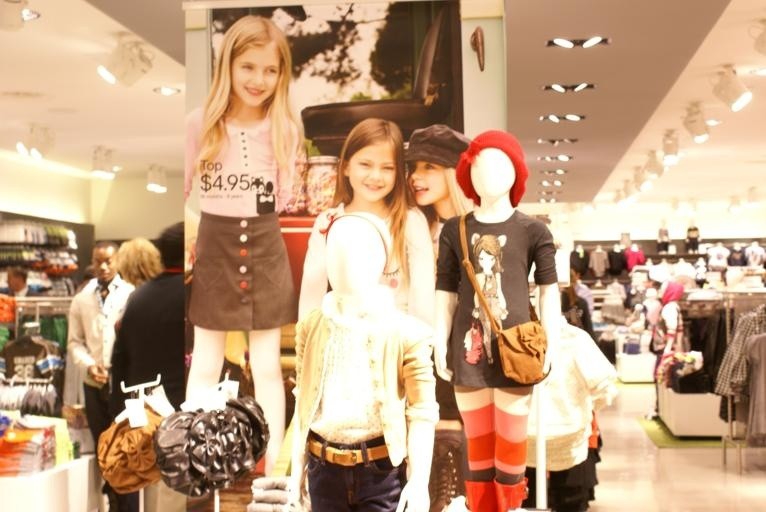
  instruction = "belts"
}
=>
[306,434,389,468]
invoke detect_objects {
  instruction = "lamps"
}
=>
[606,62,755,203]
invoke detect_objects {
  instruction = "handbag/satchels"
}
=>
[497,317,551,384]
[154,395,269,498]
[96,405,162,494]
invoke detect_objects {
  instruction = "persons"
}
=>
[0,263,44,296]
[564,216,766,365]
[294,116,437,332]
[649,278,687,417]
[180,11,310,479]
[424,129,557,511]
[276,210,443,511]
[401,117,475,511]
[68,220,191,511]
[516,251,625,511]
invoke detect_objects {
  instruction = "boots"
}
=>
[465,478,529,512]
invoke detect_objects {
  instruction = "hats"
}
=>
[402,125,470,168]
[457,131,528,208]
